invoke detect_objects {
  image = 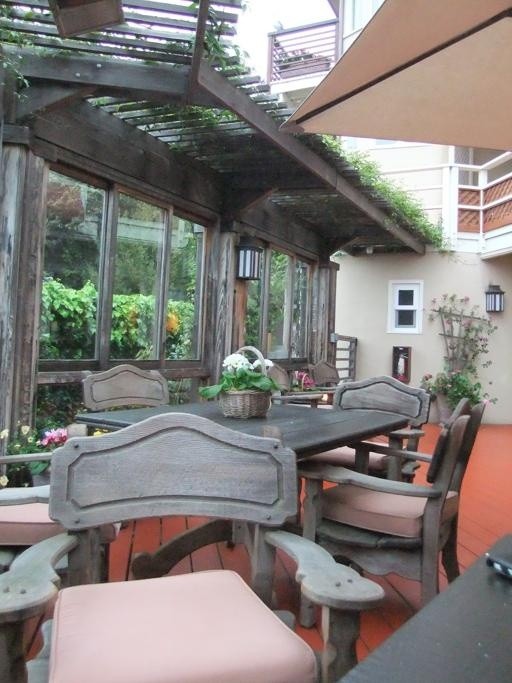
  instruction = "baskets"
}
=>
[221,346,271,418]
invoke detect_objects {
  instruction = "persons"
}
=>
[396,349,408,376]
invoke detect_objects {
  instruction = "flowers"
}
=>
[196,352,288,401]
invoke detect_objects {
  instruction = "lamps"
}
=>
[234,234,264,280]
[485,284,506,312]
